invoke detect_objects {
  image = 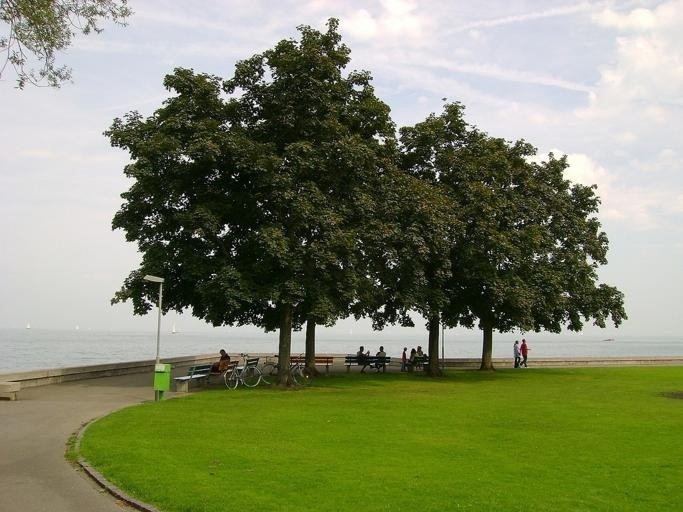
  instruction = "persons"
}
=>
[375,346,386,374]
[401,346,423,372]
[357,346,370,373]
[514,339,531,368]
[206,349,230,383]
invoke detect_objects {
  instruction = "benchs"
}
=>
[289,356,334,371]
[173,358,259,393]
[399,356,428,370]
[344,354,391,373]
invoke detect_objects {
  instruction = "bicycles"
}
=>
[222,353,261,391]
[259,353,312,386]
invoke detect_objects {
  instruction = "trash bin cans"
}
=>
[154,364,170,401]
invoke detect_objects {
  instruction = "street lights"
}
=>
[140,273,163,401]
